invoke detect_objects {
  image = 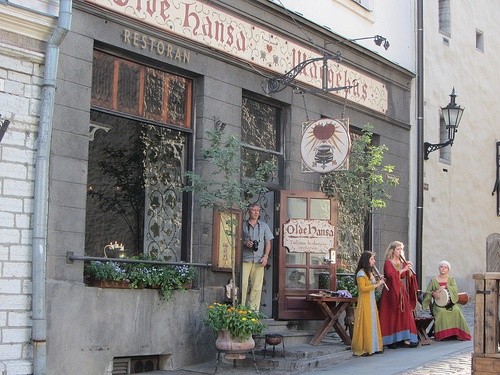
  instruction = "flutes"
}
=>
[373,264,389,291]
[400,254,416,275]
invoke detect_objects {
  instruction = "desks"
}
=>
[305,297,357,346]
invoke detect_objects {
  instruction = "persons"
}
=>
[351,251,386,356]
[378,241,419,348]
[241,204,274,314]
[422,260,472,341]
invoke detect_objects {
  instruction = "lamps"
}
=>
[424,87,465,160]
[325,34,390,50]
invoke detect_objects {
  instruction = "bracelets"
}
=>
[264,255,268,259]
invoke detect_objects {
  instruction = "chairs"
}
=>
[413,309,435,346]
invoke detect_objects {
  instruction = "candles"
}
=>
[109,242,114,249]
[114,241,119,249]
[120,244,125,250]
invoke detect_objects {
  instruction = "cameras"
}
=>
[252,240,260,251]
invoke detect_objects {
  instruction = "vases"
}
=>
[91,279,130,289]
[185,283,191,289]
[215,329,255,351]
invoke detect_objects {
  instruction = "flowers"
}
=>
[202,302,268,340]
[85,261,192,302]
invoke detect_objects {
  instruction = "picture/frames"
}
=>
[211,207,243,273]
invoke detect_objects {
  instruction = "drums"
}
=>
[435,288,468,307]
